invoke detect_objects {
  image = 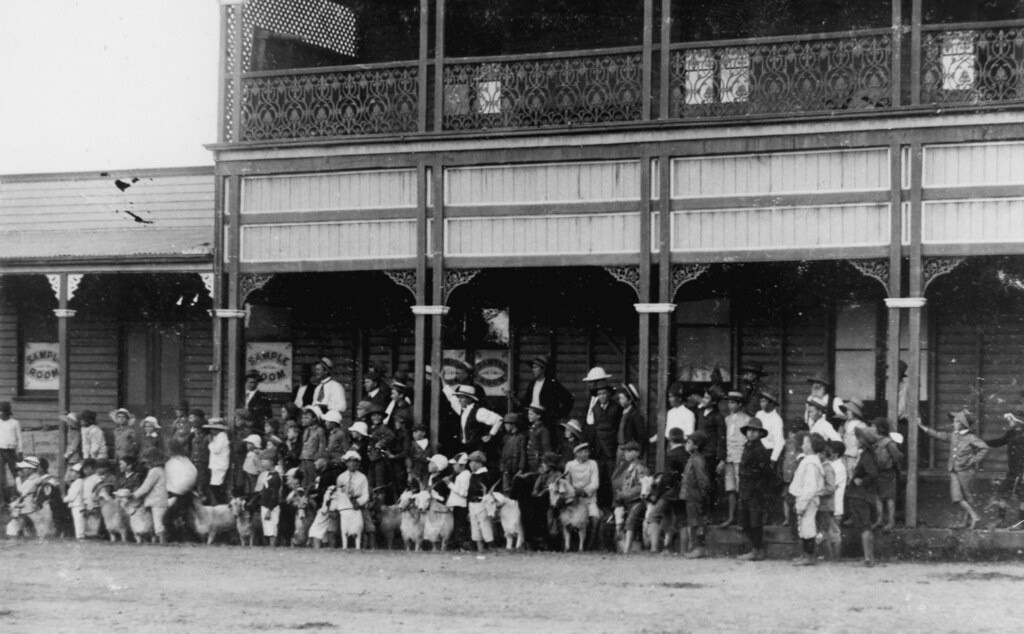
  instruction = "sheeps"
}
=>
[478,478,527,555]
[364,483,402,552]
[184,490,238,547]
[605,474,672,552]
[536,472,604,552]
[324,482,364,550]
[397,488,425,553]
[228,493,262,547]
[113,490,177,545]
[83,482,132,543]
[287,482,319,548]
[411,476,454,553]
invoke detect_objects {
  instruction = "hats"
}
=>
[947,409,975,432]
[723,391,750,405]
[760,389,780,407]
[741,359,768,376]
[807,397,826,414]
[0,402,13,415]
[867,418,889,435]
[741,419,768,438]
[855,427,878,453]
[807,369,830,385]
[16,456,40,469]
[664,383,687,403]
[897,359,907,377]
[687,432,707,448]
[60,356,641,476]
[705,384,724,406]
[839,397,865,418]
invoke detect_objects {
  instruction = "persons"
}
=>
[985,405,1024,530]
[361,360,392,408]
[662,380,727,558]
[313,356,347,412]
[723,390,786,559]
[236,367,272,420]
[501,353,574,428]
[58,410,108,541]
[423,364,453,437]
[556,419,650,556]
[581,366,647,507]
[107,407,169,546]
[231,402,302,547]
[776,369,903,566]
[885,359,909,470]
[382,370,413,427]
[410,404,561,553]
[165,398,231,545]
[281,400,414,550]
[917,407,991,530]
[436,368,504,452]
[0,400,67,545]
[739,360,770,418]
[290,359,316,407]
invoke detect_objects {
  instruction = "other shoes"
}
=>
[682,510,1023,571]
